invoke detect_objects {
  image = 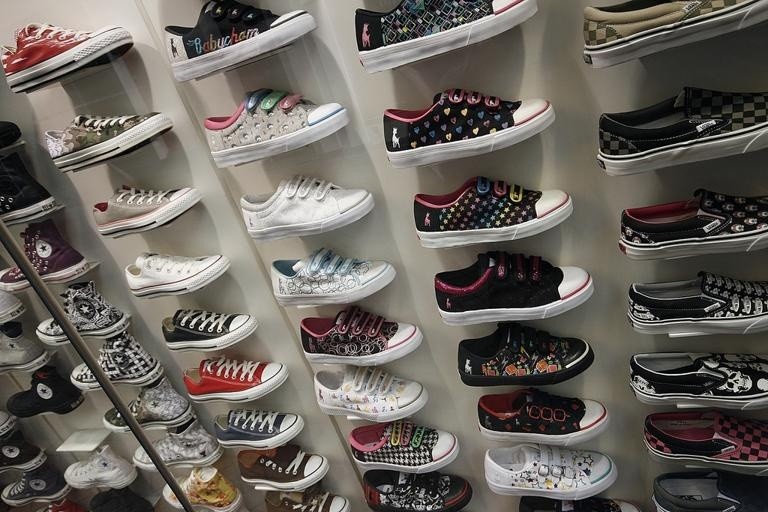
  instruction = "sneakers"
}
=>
[44,113,173,173]
[1,153,54,222]
[383,87,556,168]
[1,218,89,292]
[183,359,287,404]
[35,280,132,346]
[361,469,472,512]
[91,184,207,239]
[627,351,768,410]
[1,23,133,90]
[518,496,639,512]
[0,333,243,512]
[618,187,768,261]
[213,408,352,512]
[483,444,620,501]
[478,386,607,447]
[412,175,574,249]
[652,469,767,512]
[1,292,28,326]
[313,367,429,424]
[241,175,374,244]
[271,253,395,306]
[161,307,258,353]
[300,308,419,364]
[351,422,460,474]
[626,272,768,337]
[596,85,767,176]
[433,250,595,327]
[456,321,594,387]
[124,251,232,300]
[354,0,537,74]
[640,410,768,469]
[163,1,318,85]
[581,0,768,69]
[205,87,348,167]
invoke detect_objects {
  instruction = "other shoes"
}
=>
[1,120,22,150]
